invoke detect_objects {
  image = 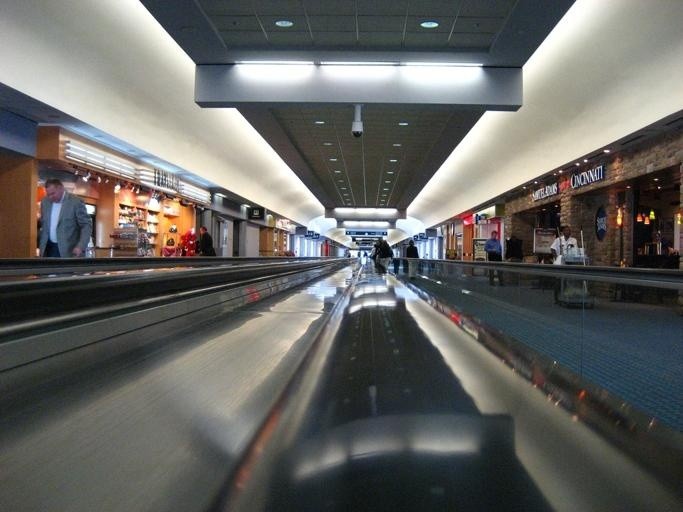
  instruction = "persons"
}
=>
[198,226,213,256]
[407,240,418,258]
[38,178,93,278]
[484,231,503,286]
[550,225,579,304]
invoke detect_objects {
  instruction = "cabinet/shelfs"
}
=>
[118,203,160,245]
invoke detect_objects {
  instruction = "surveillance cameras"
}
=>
[351,122,364,137]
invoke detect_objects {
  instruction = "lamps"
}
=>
[65,141,211,210]
[636,208,655,225]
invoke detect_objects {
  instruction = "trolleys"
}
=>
[555,248,594,308]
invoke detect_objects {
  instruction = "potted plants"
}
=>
[655,230,665,255]
